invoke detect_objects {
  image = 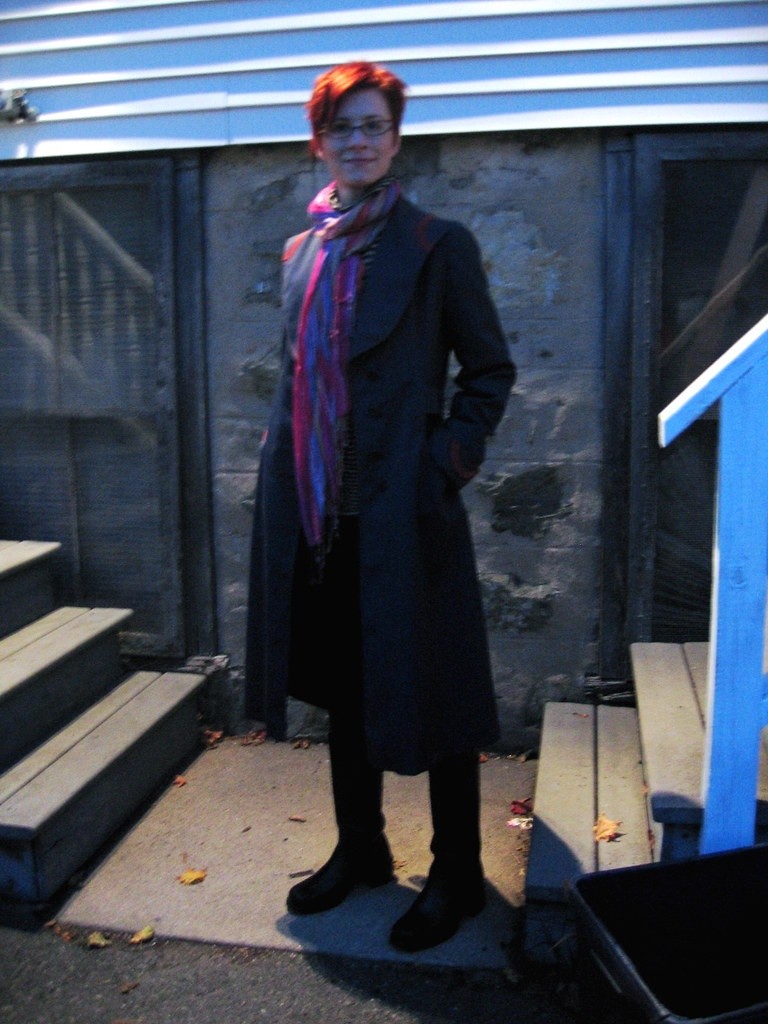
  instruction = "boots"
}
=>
[288,731,394,915]
[390,746,485,951]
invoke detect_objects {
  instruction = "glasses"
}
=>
[316,112,398,140]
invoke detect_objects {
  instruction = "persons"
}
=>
[244,64,519,955]
